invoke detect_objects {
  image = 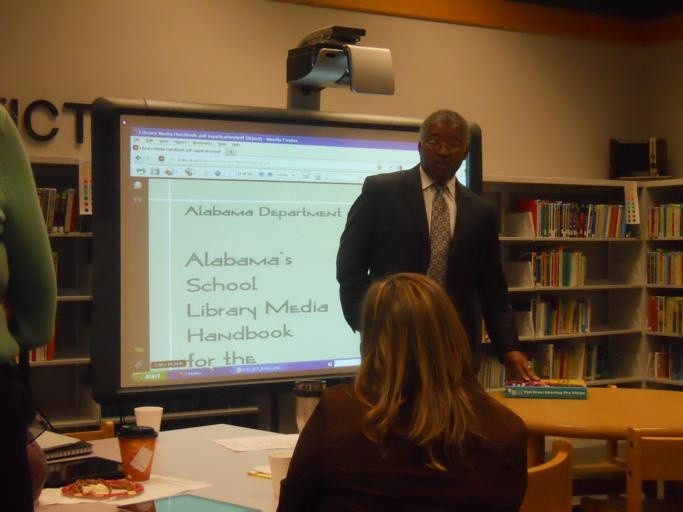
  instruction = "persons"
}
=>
[0,103,59,512]
[335,111,539,382]
[277,273,528,512]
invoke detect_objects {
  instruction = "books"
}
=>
[28,427,94,461]
[28,339,58,360]
[37,187,78,236]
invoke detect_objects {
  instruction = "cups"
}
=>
[268,449,293,506]
[291,377,329,434]
[133,406,164,437]
[114,425,159,481]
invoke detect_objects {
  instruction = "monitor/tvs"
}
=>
[89,96,482,405]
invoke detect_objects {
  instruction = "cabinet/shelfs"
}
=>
[644,179,683,392]
[472,175,645,389]
[13,157,260,430]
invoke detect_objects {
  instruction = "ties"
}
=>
[428,183,451,286]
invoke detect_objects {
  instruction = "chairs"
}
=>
[522,435,571,511]
[620,423,682,511]
[604,381,668,501]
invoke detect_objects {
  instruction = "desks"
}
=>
[482,384,683,511]
[86,423,297,511]
